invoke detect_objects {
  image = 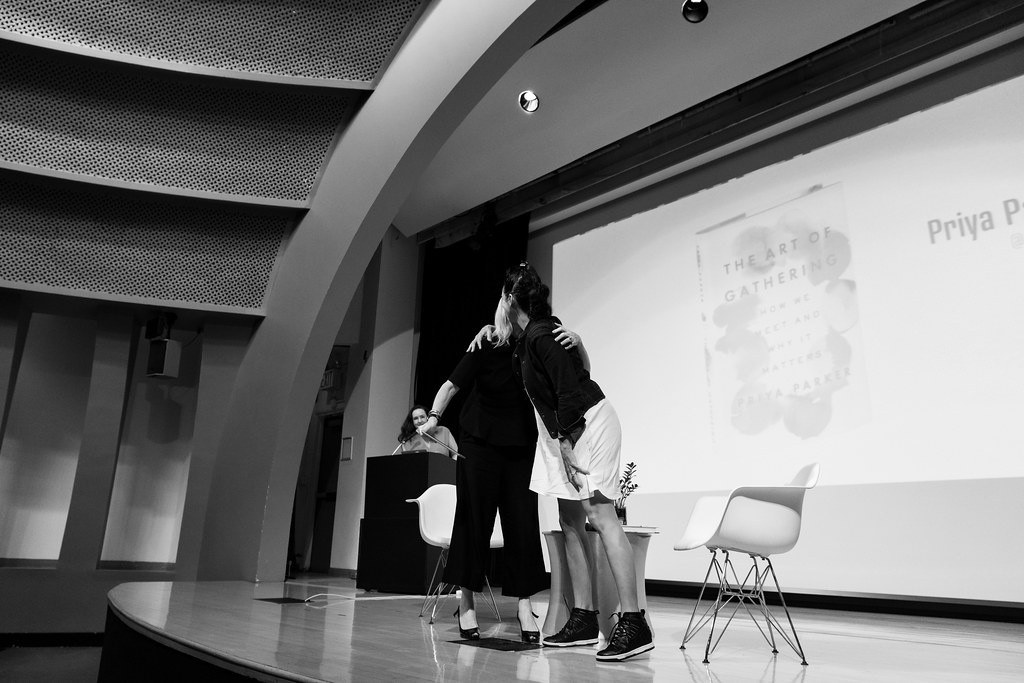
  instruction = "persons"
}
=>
[466,262,656,662]
[414,294,591,643]
[396,405,460,462]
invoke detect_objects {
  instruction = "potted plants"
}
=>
[613,461,639,525]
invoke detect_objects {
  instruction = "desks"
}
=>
[542,529,661,640]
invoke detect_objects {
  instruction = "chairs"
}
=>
[672,462,822,666]
[405,483,505,625]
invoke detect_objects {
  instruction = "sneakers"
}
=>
[596,609,655,662]
[542,607,599,647]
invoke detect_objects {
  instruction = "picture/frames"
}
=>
[340,435,353,461]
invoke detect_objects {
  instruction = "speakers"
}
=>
[145,339,181,379]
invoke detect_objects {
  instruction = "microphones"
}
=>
[402,429,419,444]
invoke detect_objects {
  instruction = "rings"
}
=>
[568,336,573,342]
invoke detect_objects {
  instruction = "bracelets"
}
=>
[427,409,441,421]
[557,436,564,442]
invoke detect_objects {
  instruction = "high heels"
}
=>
[516,610,540,643]
[453,605,479,639]
